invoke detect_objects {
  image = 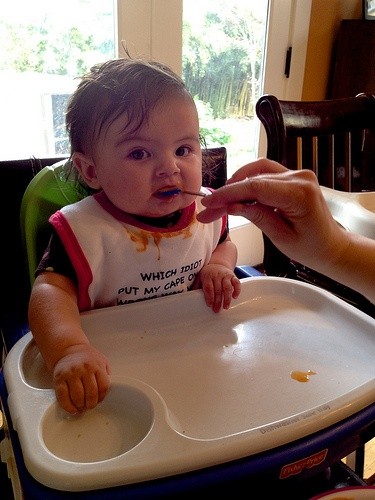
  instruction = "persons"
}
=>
[196,158,375,306]
[27,58,241,421]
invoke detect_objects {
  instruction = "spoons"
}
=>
[179,190,256,205]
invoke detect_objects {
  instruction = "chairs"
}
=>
[1,159,374,500]
[255,92,375,479]
